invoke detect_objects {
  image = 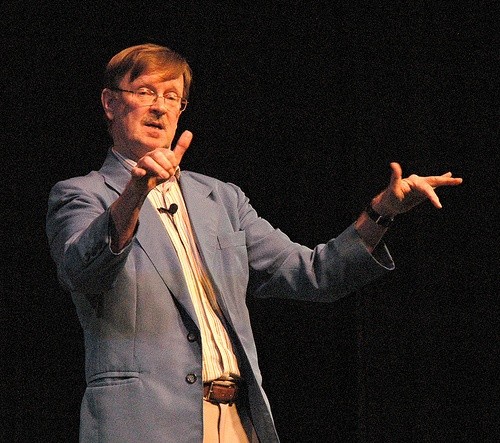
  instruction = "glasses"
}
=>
[109,87,187,112]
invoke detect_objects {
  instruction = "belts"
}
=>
[201,378,246,404]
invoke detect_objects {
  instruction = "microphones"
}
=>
[158,203,179,214]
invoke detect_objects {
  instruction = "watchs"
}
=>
[362,199,395,229]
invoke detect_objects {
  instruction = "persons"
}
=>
[43,43,466,442]
